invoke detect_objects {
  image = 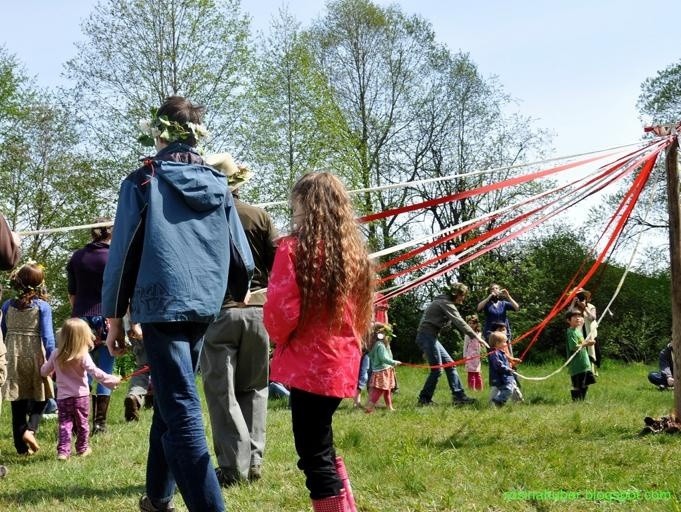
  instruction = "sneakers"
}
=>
[454,391,476,405]
[58,454,71,462]
[215,467,229,488]
[140,493,175,511]
[249,465,262,479]
[80,446,92,457]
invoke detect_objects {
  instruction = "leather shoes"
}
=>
[123,394,139,421]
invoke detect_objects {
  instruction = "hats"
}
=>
[566,287,591,302]
[205,152,256,193]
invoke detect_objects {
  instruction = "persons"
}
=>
[647,342,674,391]
[568,288,597,384]
[414,282,491,406]
[0,213,156,461]
[102,97,255,512]
[462,283,525,408]
[564,310,596,401]
[264,172,379,512]
[204,152,280,487]
[353,276,402,414]
[266,344,291,397]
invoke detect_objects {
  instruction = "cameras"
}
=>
[578,294,585,301]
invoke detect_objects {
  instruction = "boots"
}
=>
[91,395,109,435]
